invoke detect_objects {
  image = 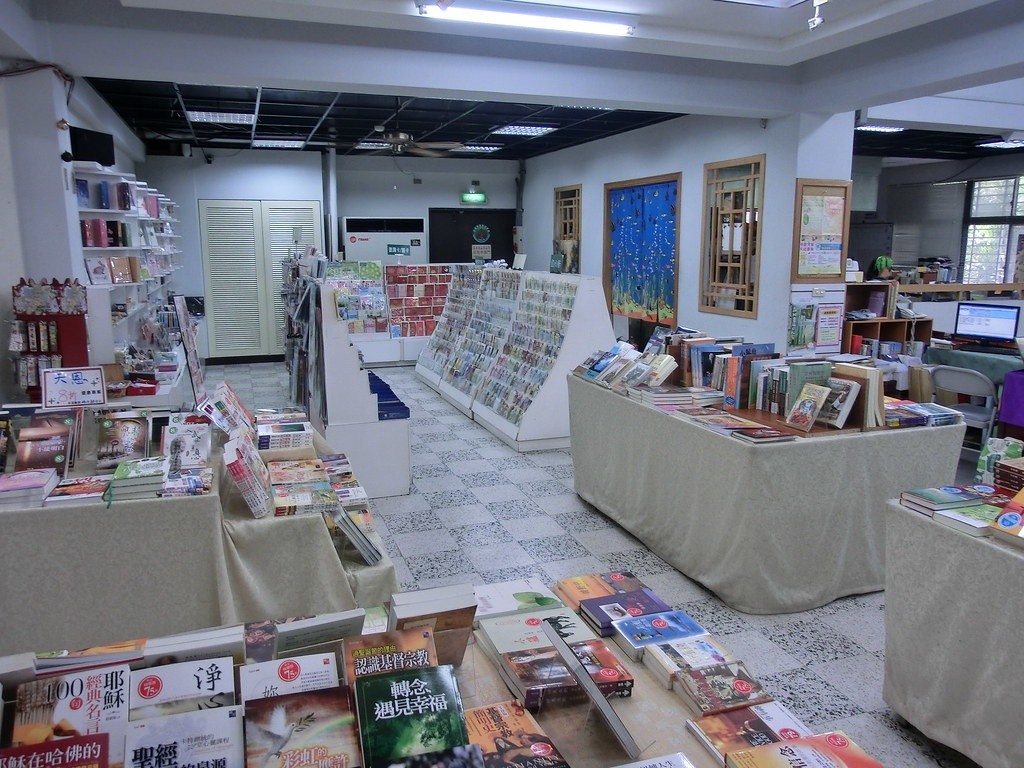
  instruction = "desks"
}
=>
[571,366,965,615]
[883,489,1024,768]
[0,580,838,768]
[929,342,1024,442]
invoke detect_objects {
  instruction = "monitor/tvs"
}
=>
[953,303,1020,347]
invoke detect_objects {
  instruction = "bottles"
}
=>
[860,340,872,356]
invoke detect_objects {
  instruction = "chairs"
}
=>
[930,367,999,460]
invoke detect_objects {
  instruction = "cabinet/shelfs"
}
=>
[1,161,620,661]
[838,282,934,383]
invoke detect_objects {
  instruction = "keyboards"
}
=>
[960,344,1021,356]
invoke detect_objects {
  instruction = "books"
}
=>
[0,166,1024,567]
[0,571,885,768]
